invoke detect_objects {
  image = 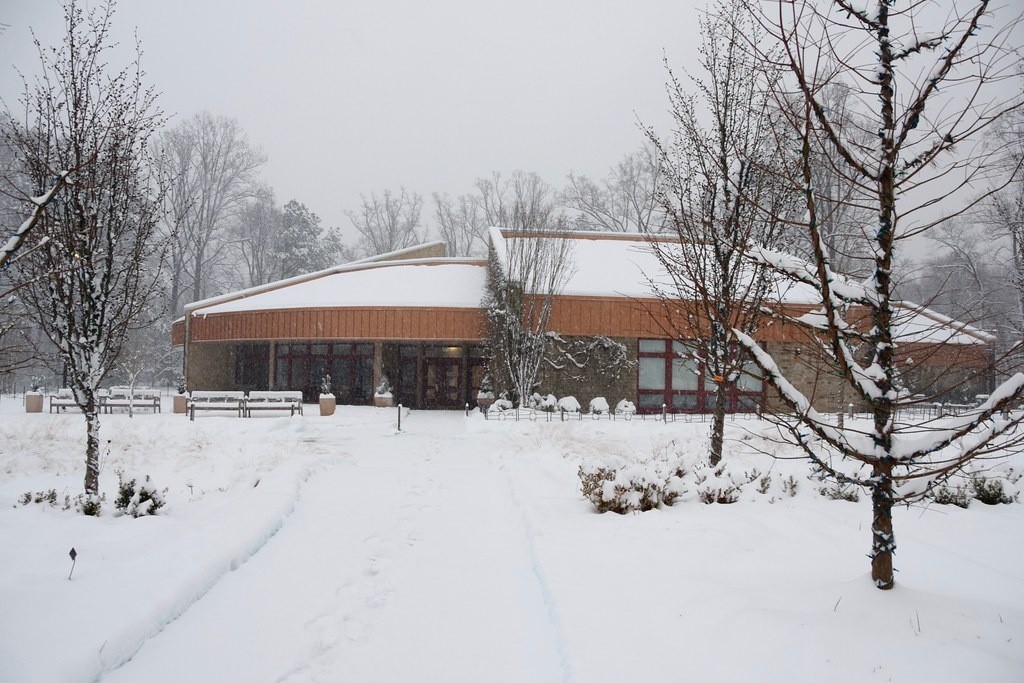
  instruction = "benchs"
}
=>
[105,389,160,415]
[50,389,108,414]
[186,391,245,417]
[246,391,303,418]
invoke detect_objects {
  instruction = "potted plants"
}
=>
[477,375,494,410]
[25,385,43,413]
[173,385,189,413]
[375,375,392,406]
[320,375,335,415]
